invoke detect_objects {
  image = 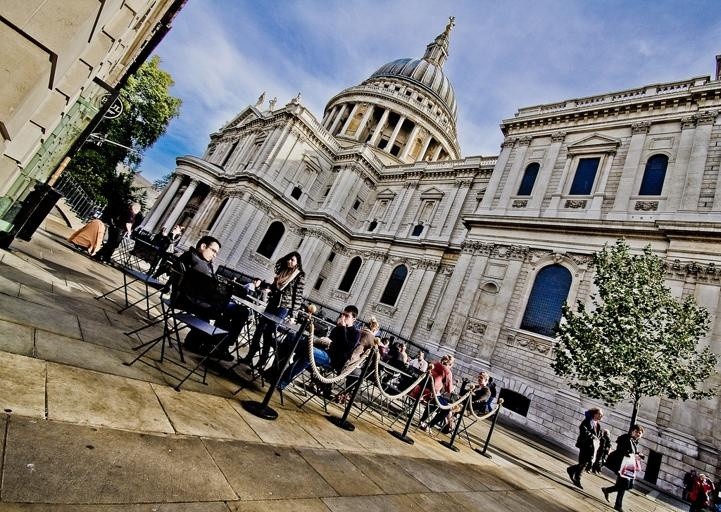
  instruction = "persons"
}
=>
[353,317,428,384]
[68,219,106,257]
[412,354,497,435]
[95,202,141,263]
[264,301,359,390]
[683,470,715,512]
[567,409,645,512]
[144,224,306,378]
[244,278,261,296]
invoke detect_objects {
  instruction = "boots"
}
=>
[236,341,271,375]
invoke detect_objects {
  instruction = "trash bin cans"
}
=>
[10,180,65,242]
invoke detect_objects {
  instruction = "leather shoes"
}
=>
[601,487,611,503]
[566,465,584,489]
[186,337,234,362]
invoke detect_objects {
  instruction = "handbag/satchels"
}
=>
[619,453,641,481]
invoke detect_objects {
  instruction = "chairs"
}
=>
[119,258,189,363]
[121,266,232,392]
[94,238,170,321]
[233,272,490,448]
